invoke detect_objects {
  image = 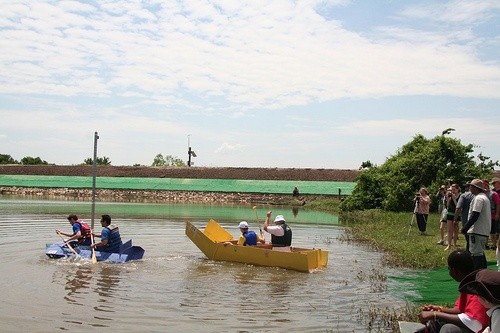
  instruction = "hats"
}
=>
[467,178,486,191]
[463,180,472,186]
[458,269,500,306]
[482,180,490,187]
[235,220,248,228]
[489,177,500,184]
[273,215,286,223]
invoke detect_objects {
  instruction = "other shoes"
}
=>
[446,240,448,245]
[444,246,451,251]
[491,247,496,250]
[421,231,426,235]
[436,240,444,245]
[486,245,489,249]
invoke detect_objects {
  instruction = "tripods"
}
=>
[408,199,435,235]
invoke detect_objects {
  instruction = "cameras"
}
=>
[414,194,420,200]
[448,192,452,197]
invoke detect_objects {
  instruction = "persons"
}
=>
[461,180,491,269]
[436,177,500,268]
[223,221,265,247]
[56,214,95,247]
[418,250,490,333]
[90,215,123,248]
[414,188,430,235]
[458,268,500,333]
[293,185,299,197]
[265,211,292,247]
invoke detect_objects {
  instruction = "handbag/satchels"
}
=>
[439,208,448,223]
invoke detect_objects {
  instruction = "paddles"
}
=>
[253,206,265,240]
[270,211,272,226]
[90,230,97,263]
[58,231,77,254]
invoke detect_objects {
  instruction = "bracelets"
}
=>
[434,311,437,319]
[440,305,443,311]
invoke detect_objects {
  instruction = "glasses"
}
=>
[465,185,470,188]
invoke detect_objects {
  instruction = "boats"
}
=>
[185,218,328,273]
[45,239,146,263]
